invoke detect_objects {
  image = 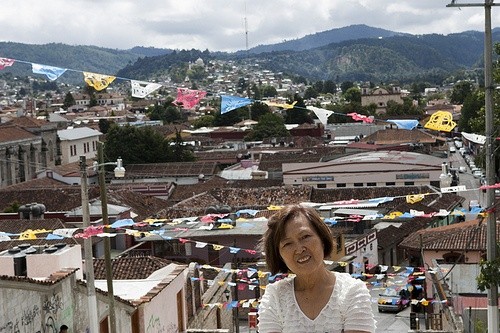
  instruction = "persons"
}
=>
[255,203,378,333]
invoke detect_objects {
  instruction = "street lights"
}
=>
[79,155,125,333]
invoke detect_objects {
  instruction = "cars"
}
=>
[377,285,407,312]
[448,136,500,197]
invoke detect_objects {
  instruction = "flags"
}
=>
[0,57,17,71]
[347,113,375,125]
[171,88,208,111]
[299,200,323,209]
[220,94,253,116]
[307,106,334,126]
[424,110,456,133]
[32,62,68,81]
[323,183,500,309]
[130,80,164,99]
[386,118,420,132]
[82,72,118,92]
[261,100,298,111]
[0,204,290,317]
[460,131,488,145]
